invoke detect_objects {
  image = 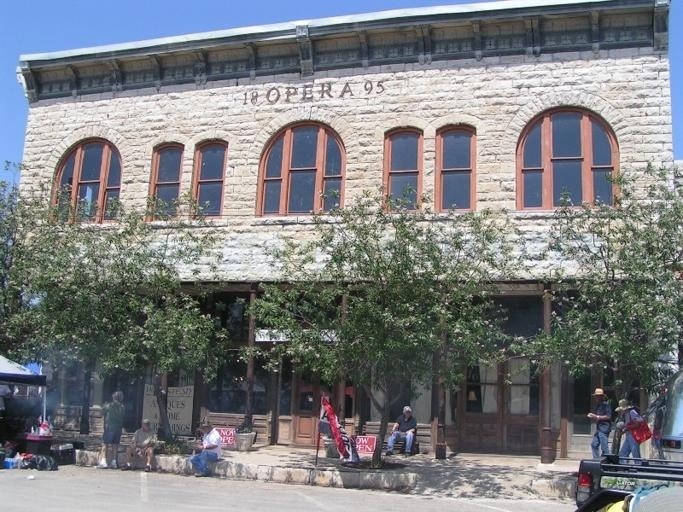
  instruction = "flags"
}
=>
[319,397,361,464]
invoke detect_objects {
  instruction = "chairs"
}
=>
[383,428,419,455]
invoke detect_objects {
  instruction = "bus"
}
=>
[647,368,682,463]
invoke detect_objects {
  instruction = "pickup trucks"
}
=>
[573,453,682,512]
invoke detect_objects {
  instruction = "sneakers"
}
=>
[95,464,131,470]
[194,471,207,476]
[144,464,151,472]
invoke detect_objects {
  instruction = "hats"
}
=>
[615,399,635,412]
[591,388,607,398]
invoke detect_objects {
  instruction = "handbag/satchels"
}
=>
[628,420,652,444]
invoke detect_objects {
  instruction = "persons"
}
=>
[616,399,644,465]
[589,388,612,458]
[121,419,156,472]
[95,391,126,469]
[191,420,222,477]
[386,406,417,457]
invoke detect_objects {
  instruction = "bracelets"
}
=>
[597,416,600,421]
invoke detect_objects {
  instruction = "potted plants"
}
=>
[234,424,255,450]
